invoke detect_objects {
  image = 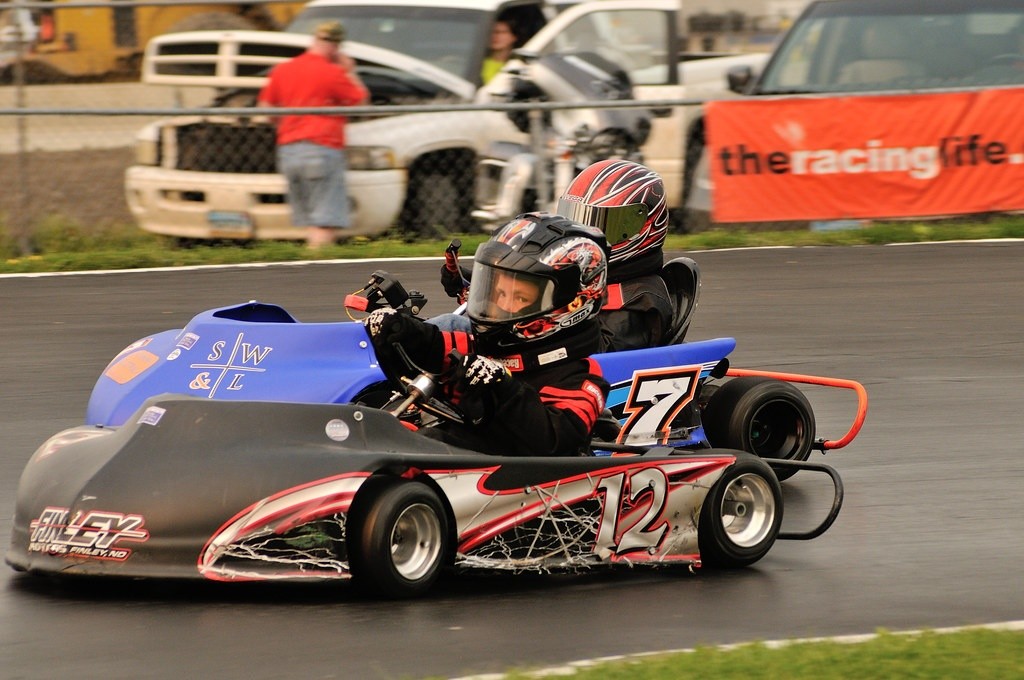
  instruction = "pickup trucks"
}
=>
[121,1,770,247]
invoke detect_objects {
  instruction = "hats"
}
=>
[310,21,347,40]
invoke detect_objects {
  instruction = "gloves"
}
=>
[441,264,473,297]
[464,355,520,405]
[364,307,412,346]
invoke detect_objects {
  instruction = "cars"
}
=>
[726,0,1024,95]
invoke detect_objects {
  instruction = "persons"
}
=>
[364,213,611,457]
[481,19,517,85]
[251,21,370,246]
[441,158,674,353]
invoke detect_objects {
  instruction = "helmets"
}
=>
[467,212,607,356]
[556,159,668,266]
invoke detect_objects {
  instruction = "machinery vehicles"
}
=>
[2,2,312,84]
